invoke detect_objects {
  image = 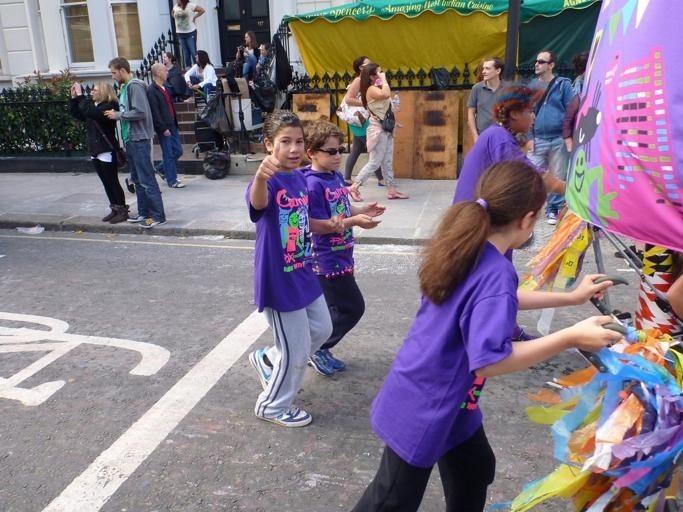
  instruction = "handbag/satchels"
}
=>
[115,149,128,169]
[336,95,370,129]
[203,149,231,180]
[382,110,396,133]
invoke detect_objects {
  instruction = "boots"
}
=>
[103,204,128,224]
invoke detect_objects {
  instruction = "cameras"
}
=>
[238,44,244,56]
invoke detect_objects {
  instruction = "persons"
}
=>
[340,57,387,192]
[467,58,510,144]
[517,50,591,224]
[70,82,130,225]
[303,119,386,377]
[245,110,338,428]
[104,57,166,228]
[148,62,188,190]
[346,61,409,203]
[342,161,621,511]
[155,0,277,135]
[449,83,575,341]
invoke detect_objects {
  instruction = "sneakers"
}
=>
[378,181,409,199]
[344,180,363,202]
[255,407,313,428]
[125,178,135,194]
[307,348,346,377]
[126,216,159,229]
[546,212,558,225]
[153,168,167,184]
[249,345,274,392]
[170,182,184,188]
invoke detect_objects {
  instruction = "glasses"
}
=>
[314,146,346,156]
[535,60,550,65]
